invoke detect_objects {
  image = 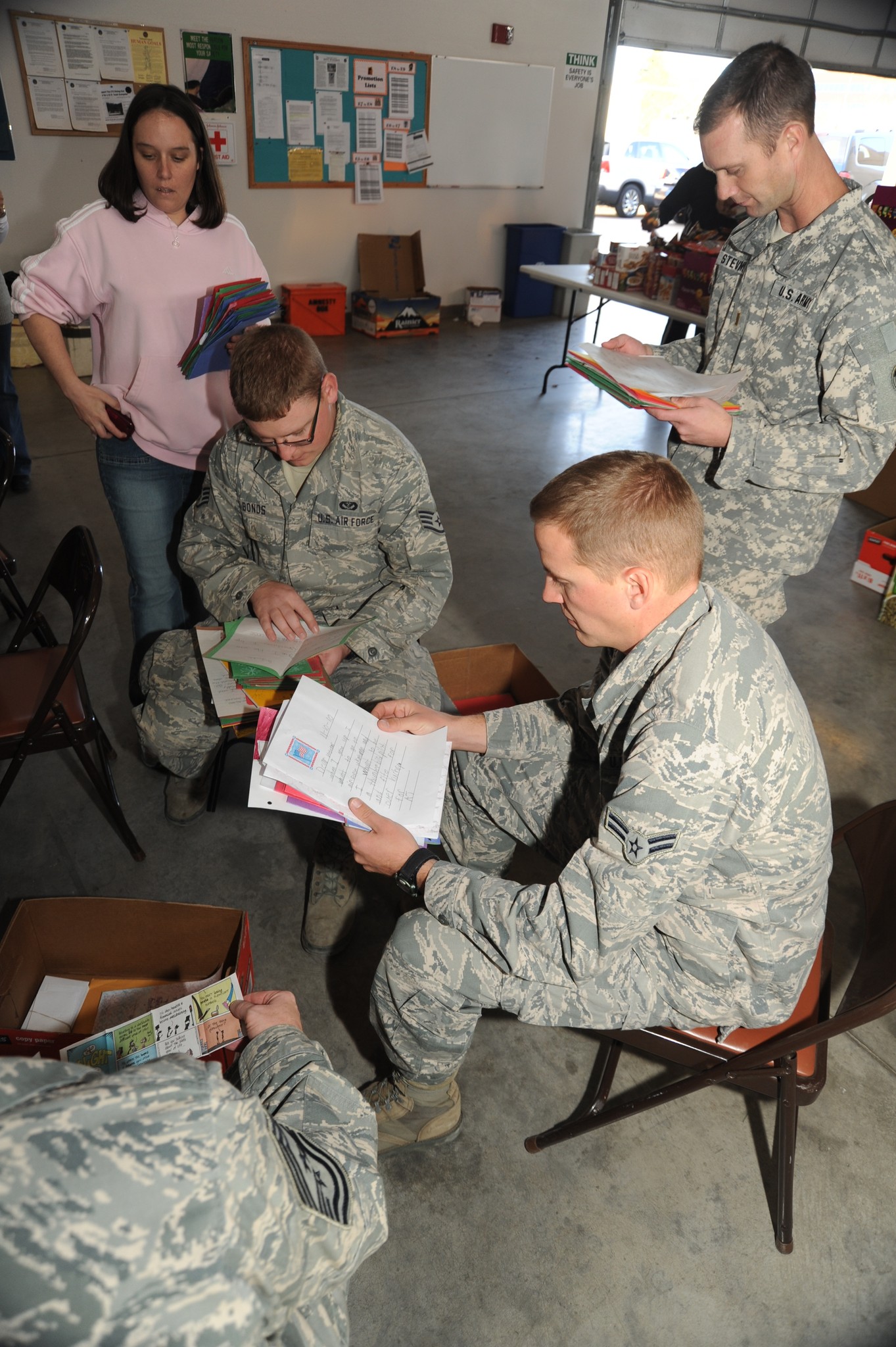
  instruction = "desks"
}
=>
[516,260,711,394]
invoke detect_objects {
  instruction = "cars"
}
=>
[598,140,698,218]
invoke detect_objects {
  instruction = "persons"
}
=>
[1,991,390,1347]
[129,323,452,951]
[637,162,746,346]
[602,40,894,632]
[6,83,278,821]
[342,448,835,1165]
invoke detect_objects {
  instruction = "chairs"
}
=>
[525,799,896,1264]
[1,524,146,864]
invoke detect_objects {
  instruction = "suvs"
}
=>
[815,129,894,209]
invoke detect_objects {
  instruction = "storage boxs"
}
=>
[588,239,722,317]
[463,284,503,325]
[281,279,348,340]
[423,642,560,724]
[849,516,896,598]
[1,897,260,1094]
[350,230,444,341]
[4,317,49,368]
[54,324,97,377]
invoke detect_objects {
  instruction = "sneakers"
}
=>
[301,831,360,956]
[359,1071,463,1154]
[164,774,213,825]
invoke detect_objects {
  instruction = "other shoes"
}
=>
[11,476,31,492]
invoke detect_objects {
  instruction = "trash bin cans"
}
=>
[551,228,601,318]
[502,223,567,318]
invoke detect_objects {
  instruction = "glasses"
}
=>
[242,373,324,447]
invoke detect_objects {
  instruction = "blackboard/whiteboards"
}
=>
[425,55,555,188]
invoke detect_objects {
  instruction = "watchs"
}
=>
[396,846,441,901]
